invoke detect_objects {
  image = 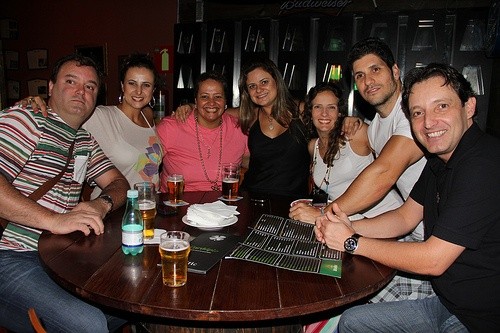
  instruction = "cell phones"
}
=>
[156,203,177,217]
[249,194,272,203]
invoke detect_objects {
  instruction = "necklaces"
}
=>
[194,108,223,191]
[310,134,332,205]
[260,108,274,130]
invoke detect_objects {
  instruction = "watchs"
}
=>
[99,194,114,214]
[344,233,363,255]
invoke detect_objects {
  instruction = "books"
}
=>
[155,231,246,274]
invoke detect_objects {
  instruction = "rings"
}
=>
[28,98,35,104]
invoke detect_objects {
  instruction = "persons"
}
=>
[306,81,405,222]
[289,39,437,304]
[13,55,164,198]
[171,57,363,197]
[0,55,131,333]
[153,72,251,192]
[314,63,500,333]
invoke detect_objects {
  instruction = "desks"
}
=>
[38,190,396,333]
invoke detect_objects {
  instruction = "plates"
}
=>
[290,199,332,210]
[182,215,238,231]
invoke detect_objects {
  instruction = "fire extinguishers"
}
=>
[155,80,169,125]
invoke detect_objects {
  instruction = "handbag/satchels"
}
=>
[308,183,328,204]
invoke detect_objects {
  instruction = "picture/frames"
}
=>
[75,42,108,76]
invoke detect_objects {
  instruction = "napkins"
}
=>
[187,200,241,226]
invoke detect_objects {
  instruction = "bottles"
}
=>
[121,190,144,255]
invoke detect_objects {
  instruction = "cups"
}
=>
[166,174,184,205]
[222,162,240,200]
[159,230,190,287]
[133,182,157,236]
